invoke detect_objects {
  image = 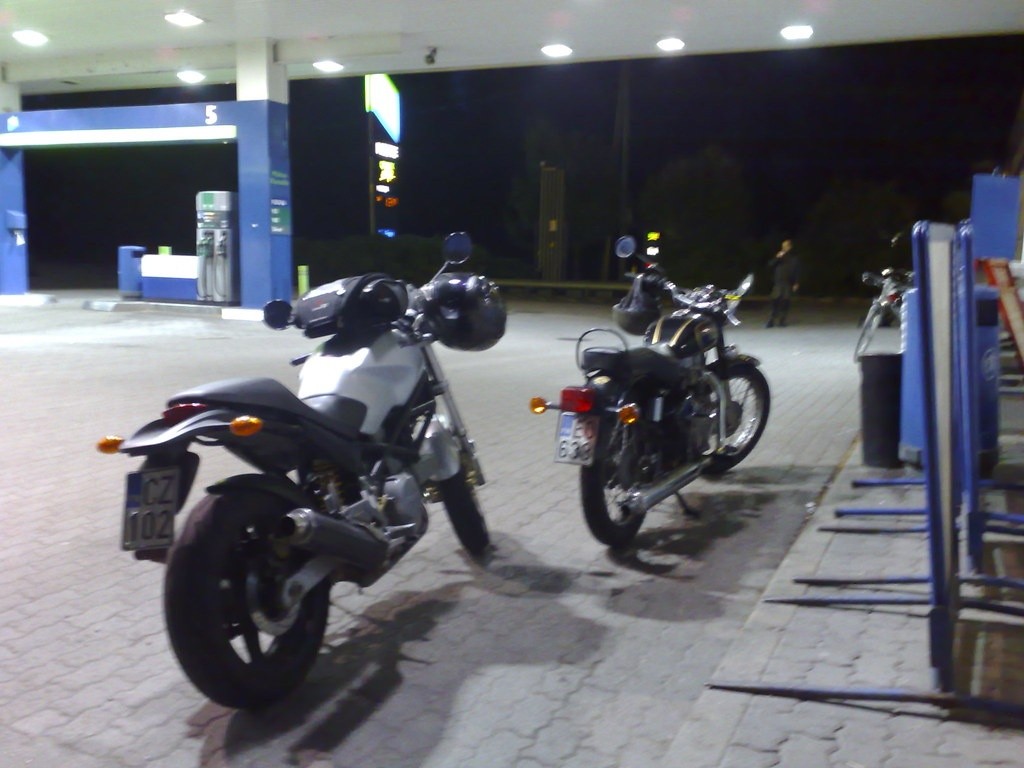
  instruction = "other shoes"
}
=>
[780,320,786,327]
[764,321,774,327]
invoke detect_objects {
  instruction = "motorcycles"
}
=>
[528,235,772,547]
[97,231,491,711]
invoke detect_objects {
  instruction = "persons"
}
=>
[762,239,800,329]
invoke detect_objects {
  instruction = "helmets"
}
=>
[417,272,507,352]
[610,304,662,335]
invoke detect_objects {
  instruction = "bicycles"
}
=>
[852,269,915,362]
